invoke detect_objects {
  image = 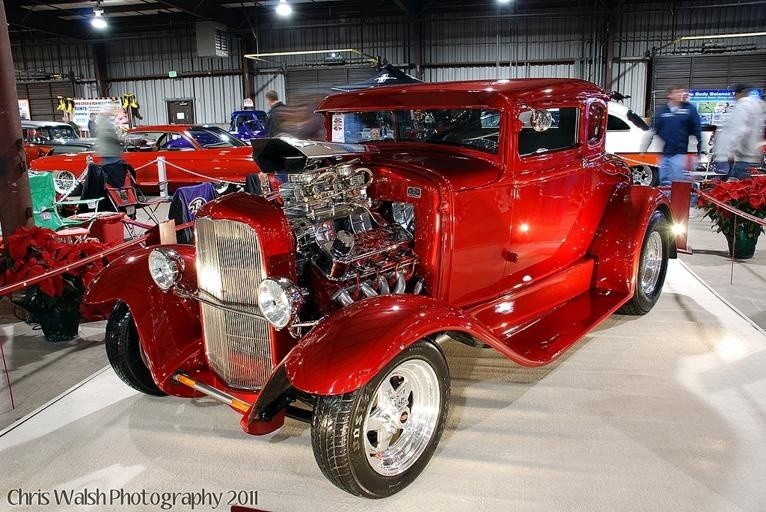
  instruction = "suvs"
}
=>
[21,120,98,156]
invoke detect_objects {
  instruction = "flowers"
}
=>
[1,223,113,298]
[696,174,766,239]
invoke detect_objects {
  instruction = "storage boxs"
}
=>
[69,212,125,244]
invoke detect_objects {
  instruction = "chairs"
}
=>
[99,170,216,242]
[28,174,90,242]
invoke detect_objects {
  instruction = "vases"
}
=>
[17,286,87,343]
[723,227,760,260]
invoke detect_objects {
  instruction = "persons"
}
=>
[636,84,704,193]
[714,81,766,182]
[92,104,126,167]
[265,90,287,111]
[87,113,96,138]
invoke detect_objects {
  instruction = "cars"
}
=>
[30,125,262,196]
